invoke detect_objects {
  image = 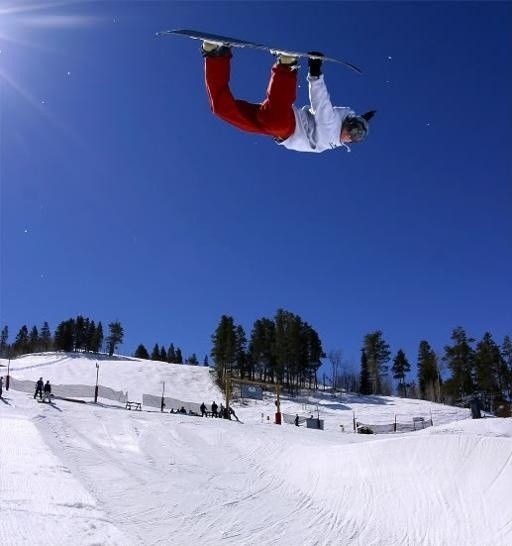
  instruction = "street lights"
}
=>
[93,363,102,403]
[158,380,168,414]
[5,351,14,391]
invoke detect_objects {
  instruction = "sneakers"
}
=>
[276,55,298,66]
[200,41,233,58]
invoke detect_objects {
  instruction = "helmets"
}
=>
[349,116,370,142]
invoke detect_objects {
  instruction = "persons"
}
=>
[42,381,52,403]
[201,39,376,153]
[170,401,238,421]
[0,376,5,399]
[34,378,44,399]
[294,414,300,426]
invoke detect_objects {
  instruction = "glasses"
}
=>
[350,121,365,142]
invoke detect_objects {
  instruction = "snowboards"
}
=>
[38,401,56,405]
[156,29,365,76]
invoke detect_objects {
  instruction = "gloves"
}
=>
[307,51,323,75]
[361,111,376,122]
